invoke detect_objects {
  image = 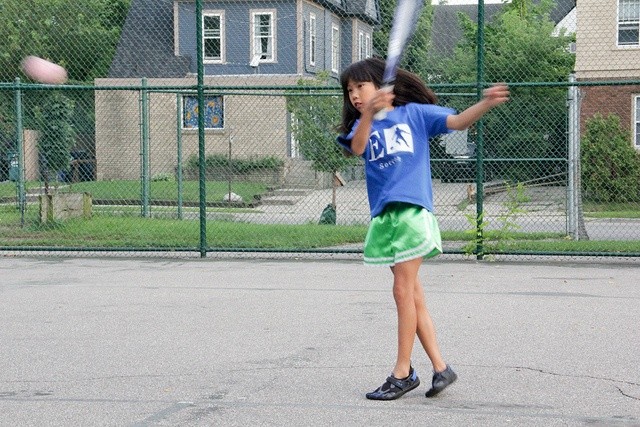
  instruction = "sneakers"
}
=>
[366,365,421,400]
[425,362,457,396]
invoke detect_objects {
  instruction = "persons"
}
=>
[337,56,510,401]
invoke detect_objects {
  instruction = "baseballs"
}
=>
[22,56,67,84]
[373,0,422,120]
